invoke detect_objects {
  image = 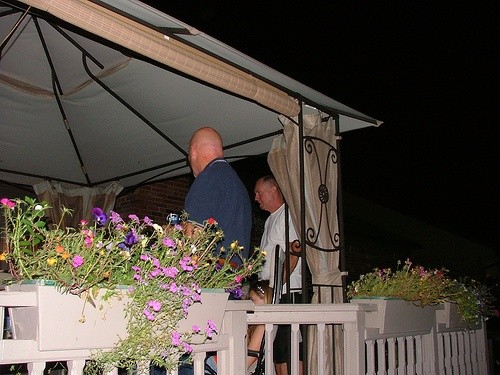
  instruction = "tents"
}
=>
[0,0,385,304]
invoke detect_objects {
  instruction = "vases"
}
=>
[350,299,436,335]
[435,303,474,329]
[4,278,230,352]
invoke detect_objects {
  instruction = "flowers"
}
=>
[343,256,450,308]
[450,278,497,329]
[0,197,269,375]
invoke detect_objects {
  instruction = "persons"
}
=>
[150,175,303,375]
[182,127,252,275]
